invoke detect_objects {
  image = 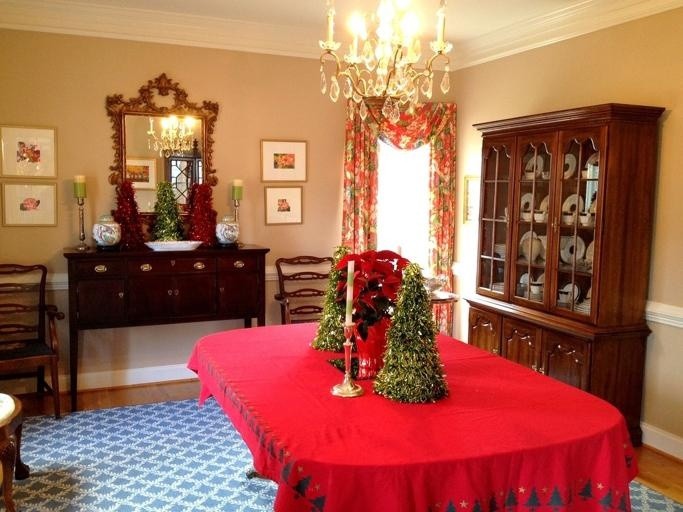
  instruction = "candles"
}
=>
[344,260,355,326]
[231,179,243,200]
[72,176,85,199]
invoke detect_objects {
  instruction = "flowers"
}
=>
[333,250,411,341]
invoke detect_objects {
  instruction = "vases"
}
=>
[352,316,393,380]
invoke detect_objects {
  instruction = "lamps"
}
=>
[317,0,453,124]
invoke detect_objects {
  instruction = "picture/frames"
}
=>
[259,138,308,226]
[462,176,480,225]
[0,123,58,228]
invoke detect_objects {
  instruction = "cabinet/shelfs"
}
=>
[461,101,665,444]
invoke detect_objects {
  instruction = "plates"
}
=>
[143,241,203,253]
[494,154,600,306]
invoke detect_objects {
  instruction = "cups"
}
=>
[520,208,593,227]
[529,280,572,303]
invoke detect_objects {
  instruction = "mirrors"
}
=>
[104,72,218,225]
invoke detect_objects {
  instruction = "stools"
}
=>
[0,393,29,511]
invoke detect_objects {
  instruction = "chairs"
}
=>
[0,264,64,424]
[274,257,334,324]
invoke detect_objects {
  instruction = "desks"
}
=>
[62,244,270,411]
[423,290,459,336]
[186,323,639,511]
